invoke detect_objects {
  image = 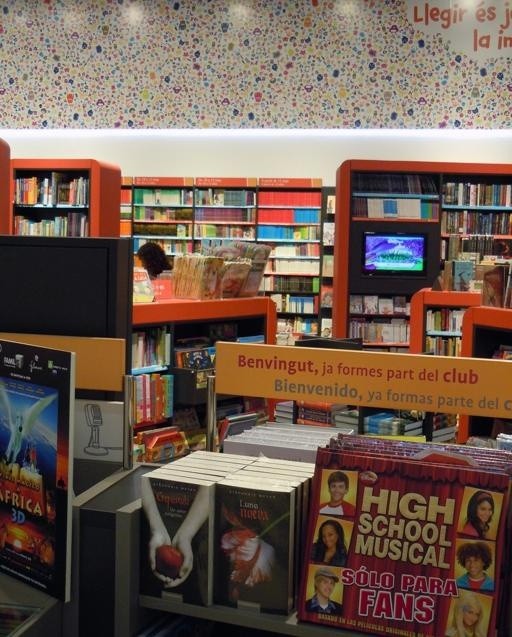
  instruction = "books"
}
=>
[0,337,80,607]
[9,167,93,239]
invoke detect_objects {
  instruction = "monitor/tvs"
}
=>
[361,231,427,280]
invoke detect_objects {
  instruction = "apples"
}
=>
[156,545,182,578]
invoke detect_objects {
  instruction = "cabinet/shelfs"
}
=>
[129,296,278,465]
[0,157,120,238]
[120,176,335,339]
[335,159,512,447]
